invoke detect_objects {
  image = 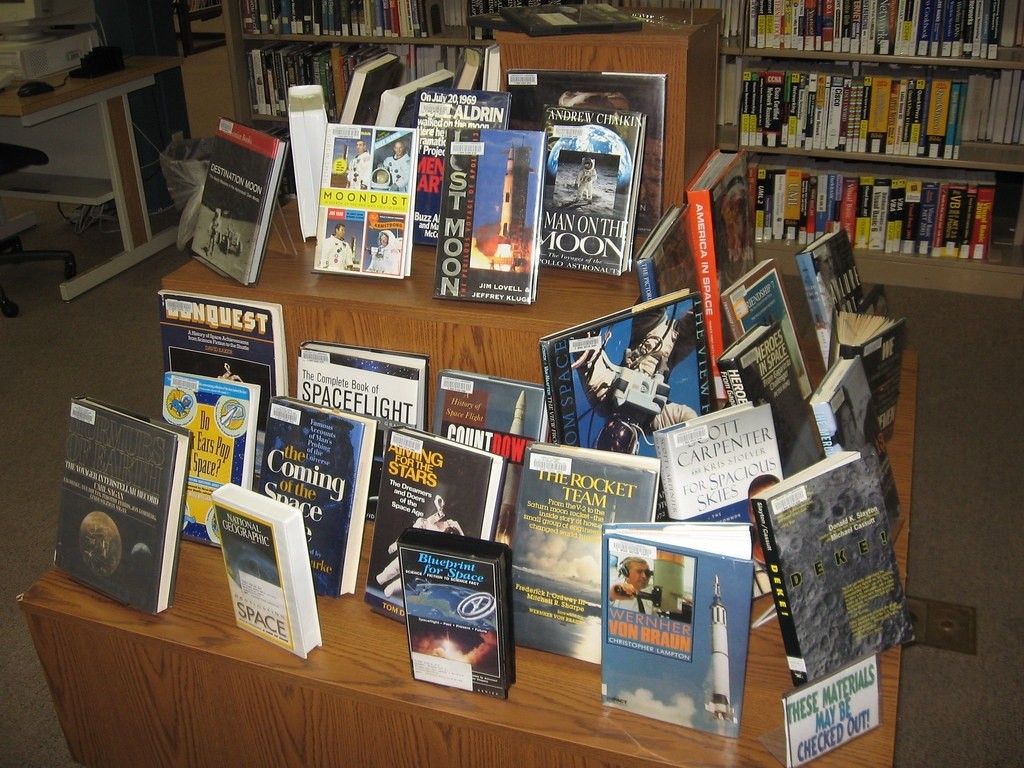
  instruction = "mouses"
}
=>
[18,82,54,97]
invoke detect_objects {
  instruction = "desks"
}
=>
[0,32,228,301]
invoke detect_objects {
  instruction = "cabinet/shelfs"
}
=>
[209,0,1024,298]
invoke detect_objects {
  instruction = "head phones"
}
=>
[617,558,632,578]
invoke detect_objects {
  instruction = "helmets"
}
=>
[582,157,595,171]
[371,168,390,189]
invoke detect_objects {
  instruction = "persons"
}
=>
[577,157,597,205]
[383,141,411,193]
[345,140,371,190]
[323,224,354,270]
[610,557,653,615]
[367,230,401,274]
[206,208,221,256]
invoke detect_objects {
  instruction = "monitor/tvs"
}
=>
[0,0,97,46]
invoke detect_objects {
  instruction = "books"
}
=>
[189,114,292,288]
[210,480,323,660]
[538,103,647,279]
[748,449,921,691]
[431,124,550,307]
[599,518,756,739]
[312,122,420,280]
[240,0,1024,262]
[158,149,906,665]
[52,394,194,617]
[396,525,519,701]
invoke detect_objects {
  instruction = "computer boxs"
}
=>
[0,27,100,79]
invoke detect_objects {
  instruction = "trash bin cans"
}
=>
[160,139,215,258]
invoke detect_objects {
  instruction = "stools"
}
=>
[0,143,76,316]
